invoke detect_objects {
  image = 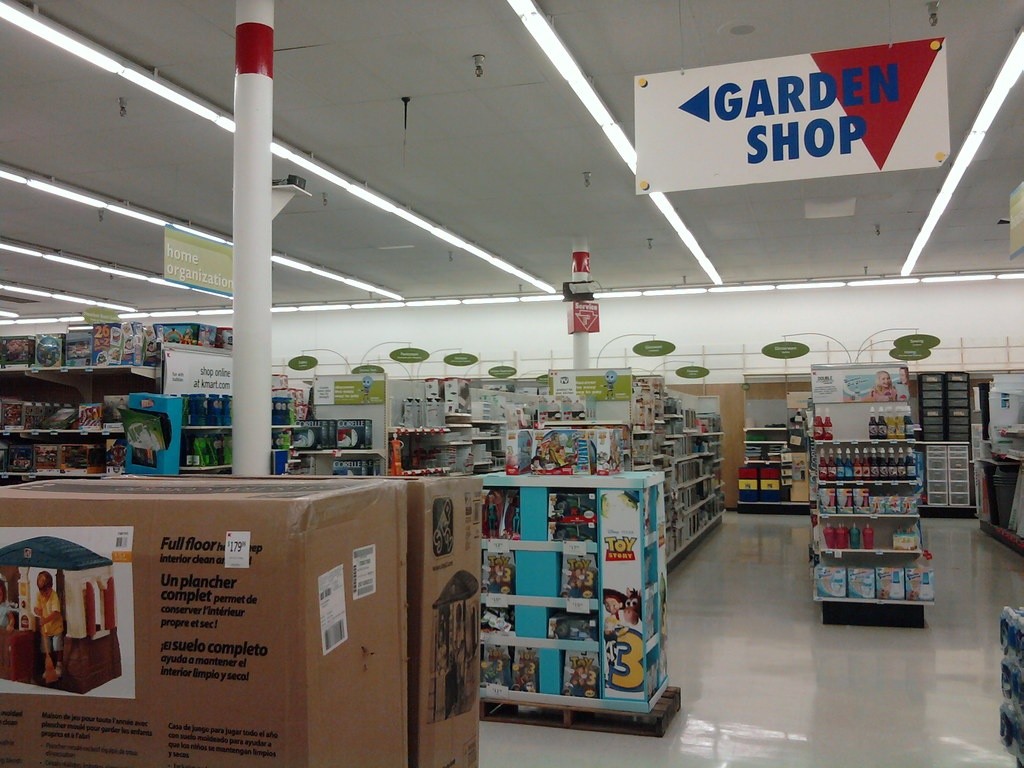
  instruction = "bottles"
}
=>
[813,406,918,549]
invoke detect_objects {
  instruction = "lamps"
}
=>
[1,0,1024,331]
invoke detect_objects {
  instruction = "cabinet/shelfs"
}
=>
[0,365,163,477]
[812,438,934,628]
[738,425,811,514]
[977,458,1024,557]
[180,414,725,572]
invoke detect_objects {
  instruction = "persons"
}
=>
[871,371,897,402]
[898,367,909,386]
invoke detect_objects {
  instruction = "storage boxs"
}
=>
[917,371,970,504]
[0,323,631,768]
[813,562,934,601]
[739,467,783,501]
